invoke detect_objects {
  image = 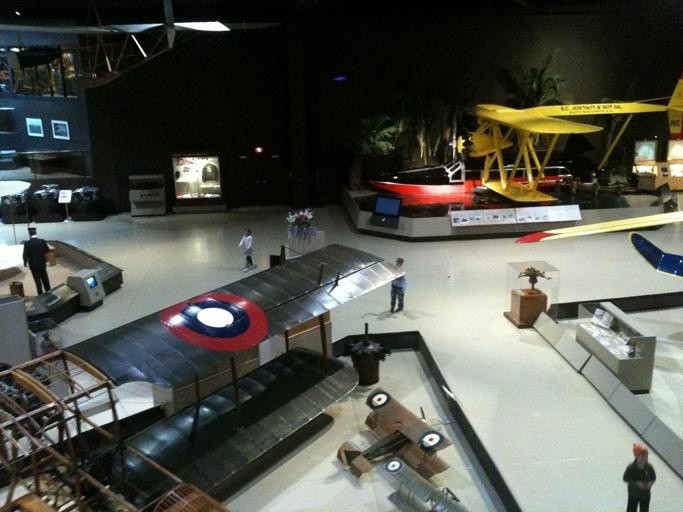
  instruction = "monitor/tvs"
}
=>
[86,275,98,289]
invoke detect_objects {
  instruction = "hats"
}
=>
[28,227,36,230]
[633,443,649,456]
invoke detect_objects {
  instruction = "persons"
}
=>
[390,257,407,311]
[22,228,50,295]
[621,443,656,511]
[236,228,255,272]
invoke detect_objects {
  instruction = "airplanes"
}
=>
[449,79,682,203]
